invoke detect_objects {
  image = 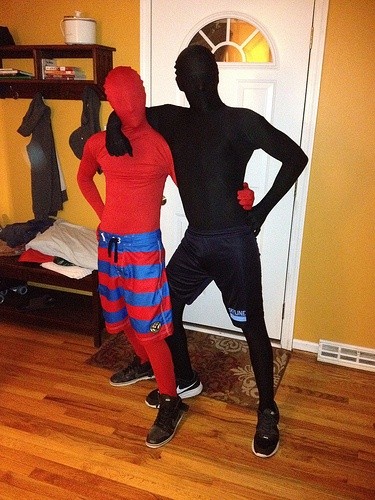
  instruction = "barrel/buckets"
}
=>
[60,11,97,45]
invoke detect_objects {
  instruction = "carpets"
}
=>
[82,330,291,410]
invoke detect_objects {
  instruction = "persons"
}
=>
[105,45,309,458]
[77,66,255,448]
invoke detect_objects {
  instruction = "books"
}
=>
[0,67,33,80]
[41,58,84,80]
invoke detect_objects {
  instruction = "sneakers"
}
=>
[145,394,184,448]
[110,356,155,387]
[252,402,280,457]
[146,373,204,408]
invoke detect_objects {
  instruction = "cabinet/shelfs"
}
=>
[0,44,116,101]
[0,255,105,347]
